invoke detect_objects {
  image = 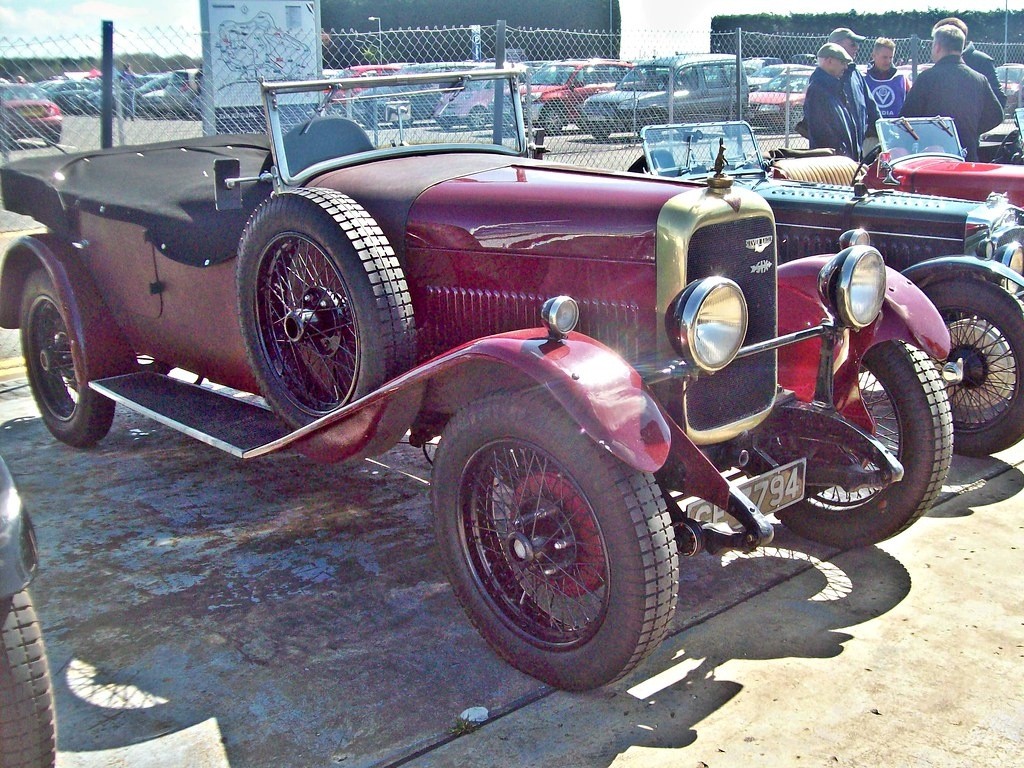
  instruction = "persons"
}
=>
[804,17,1008,163]
[119,63,136,122]
[16,75,25,84]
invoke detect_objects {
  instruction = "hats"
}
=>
[829,27,866,42]
[817,42,853,62]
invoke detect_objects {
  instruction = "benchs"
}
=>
[775,155,861,185]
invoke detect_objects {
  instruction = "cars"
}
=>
[1,51,1023,167]
[0,62,951,694]
[750,114,1024,223]
[621,119,1023,458]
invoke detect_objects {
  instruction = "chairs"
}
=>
[258,117,374,183]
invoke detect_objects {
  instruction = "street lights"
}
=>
[368,17,382,65]
